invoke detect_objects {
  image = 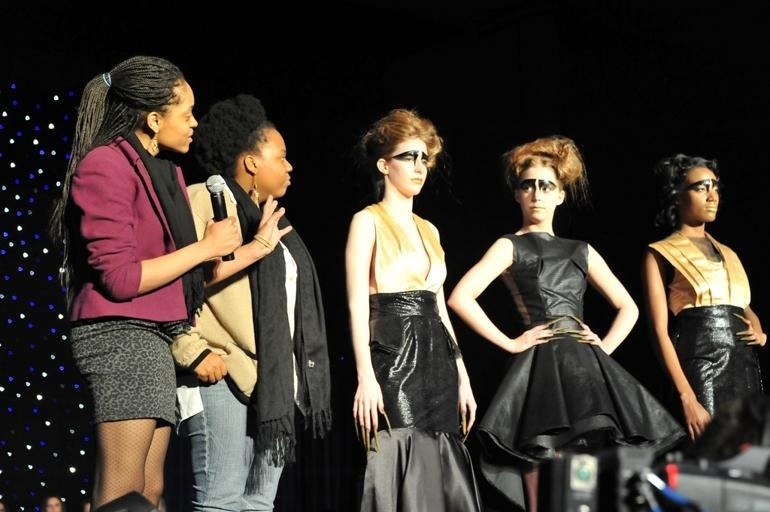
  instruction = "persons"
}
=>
[48,57,294,512]
[170,93,332,512]
[344,109,482,512]
[644,154,770,472]
[42,493,63,512]
[447,136,688,512]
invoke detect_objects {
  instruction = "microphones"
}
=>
[207,173,238,265]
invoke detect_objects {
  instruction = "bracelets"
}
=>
[253,234,275,251]
